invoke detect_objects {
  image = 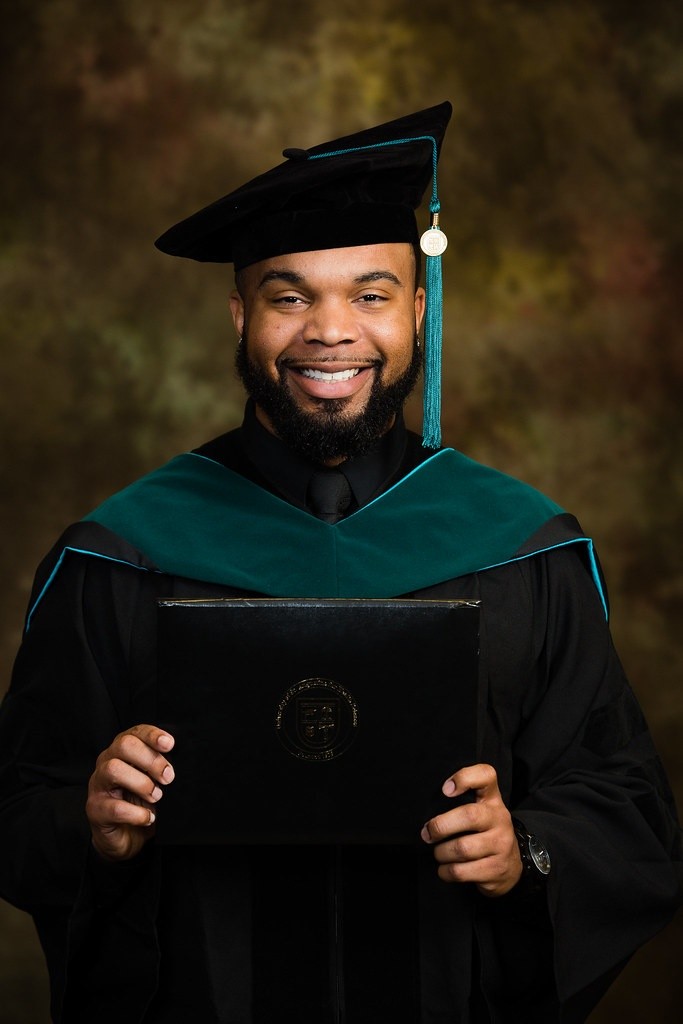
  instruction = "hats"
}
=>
[153,100,453,450]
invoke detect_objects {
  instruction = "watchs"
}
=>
[509,818,552,899]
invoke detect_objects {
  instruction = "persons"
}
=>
[0,101,683,1024]
